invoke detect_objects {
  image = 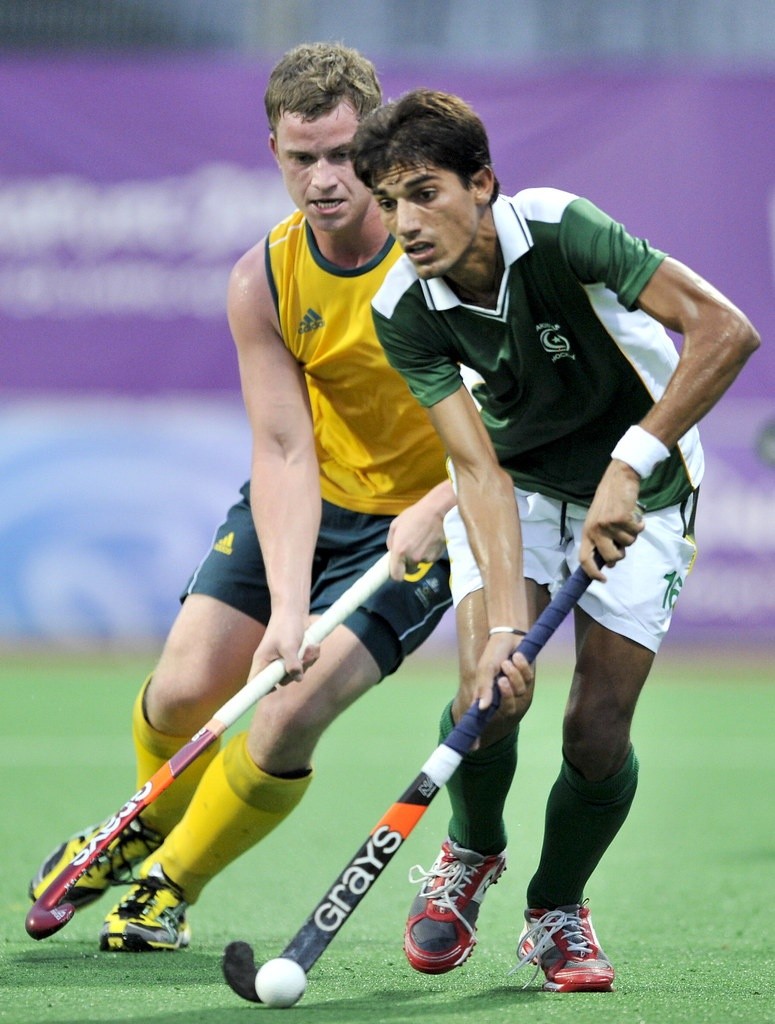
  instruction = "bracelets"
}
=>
[488,627,527,638]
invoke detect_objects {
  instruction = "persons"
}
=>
[351,91,765,987]
[25,45,454,950]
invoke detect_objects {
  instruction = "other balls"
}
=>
[253,958,308,1009]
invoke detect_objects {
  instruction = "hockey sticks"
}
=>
[220,500,650,1004]
[24,550,389,940]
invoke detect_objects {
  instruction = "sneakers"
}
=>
[505,899,616,992]
[98,862,192,951]
[402,838,506,975]
[28,814,166,909]
